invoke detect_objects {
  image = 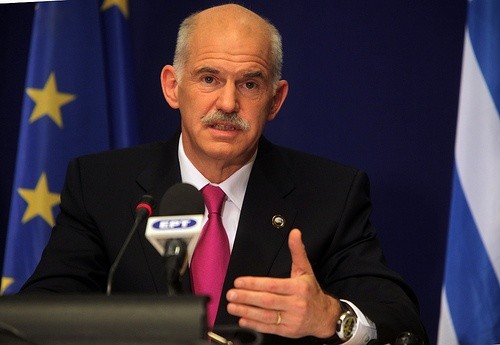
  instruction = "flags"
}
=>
[1,0,136,295]
[438,0,500,344]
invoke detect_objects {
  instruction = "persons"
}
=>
[17,3,428,345]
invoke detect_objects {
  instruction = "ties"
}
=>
[188,183,231,330]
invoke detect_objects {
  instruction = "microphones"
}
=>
[145,183,206,294]
[106,194,157,296]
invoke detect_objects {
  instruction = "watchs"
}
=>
[332,299,357,344]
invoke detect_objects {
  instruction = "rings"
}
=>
[275,311,282,325]
